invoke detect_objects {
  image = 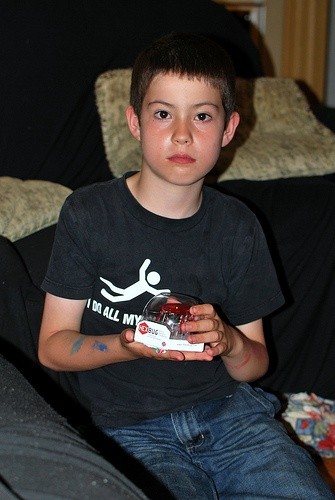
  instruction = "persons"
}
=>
[38,31,334,500]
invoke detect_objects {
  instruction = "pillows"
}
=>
[1,174,75,240]
[95,70,335,180]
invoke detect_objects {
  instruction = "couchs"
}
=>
[1,1,333,498]
